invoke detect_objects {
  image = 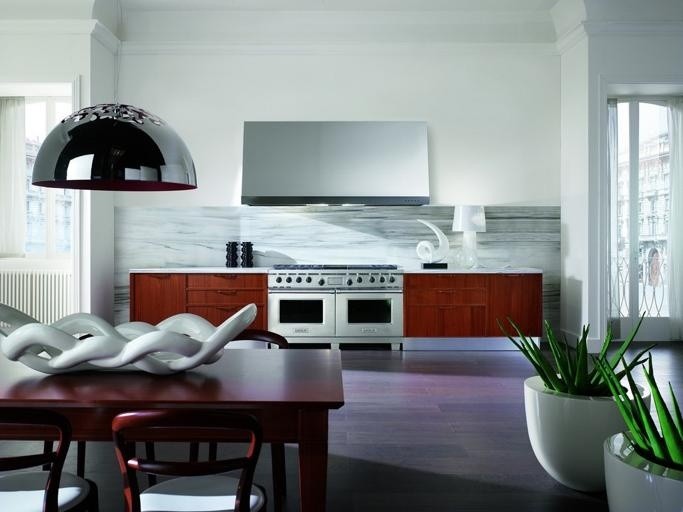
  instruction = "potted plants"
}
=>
[592,352,683,512]
[489,307,658,491]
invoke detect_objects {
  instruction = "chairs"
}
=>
[1,405,99,510]
[188,330,286,511]
[0,329,158,485]
[111,408,269,512]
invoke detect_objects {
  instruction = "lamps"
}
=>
[451,202,486,271]
[31,0,198,191]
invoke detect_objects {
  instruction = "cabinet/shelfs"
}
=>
[130,273,186,325]
[186,272,268,331]
[404,272,543,353]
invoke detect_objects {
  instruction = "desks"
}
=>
[0,349,346,512]
